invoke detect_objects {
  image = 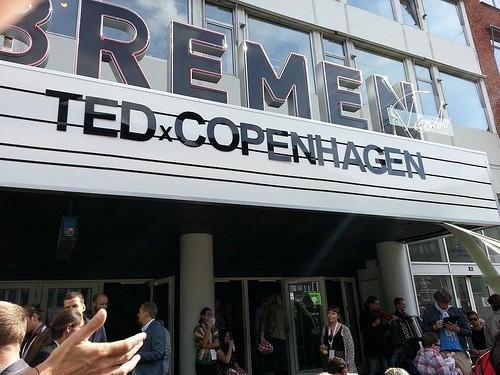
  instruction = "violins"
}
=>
[372,309,402,321]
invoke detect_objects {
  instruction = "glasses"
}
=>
[225,335,230,337]
[468,317,477,321]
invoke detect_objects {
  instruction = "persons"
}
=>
[193,307,223,375]
[215,328,238,375]
[89,293,109,320]
[257,296,290,375]
[462,293,500,375]
[421,288,473,375]
[359,296,398,375]
[389,298,444,375]
[16,307,148,375]
[254,294,270,350]
[319,304,355,375]
[215,298,239,375]
[19,303,49,364]
[131,301,166,375]
[413,334,464,375]
[24,307,84,368]
[156,320,173,375]
[0,300,33,375]
[64,291,107,343]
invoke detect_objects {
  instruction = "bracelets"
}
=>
[35,367,40,375]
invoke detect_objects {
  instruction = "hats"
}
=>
[433,288,451,303]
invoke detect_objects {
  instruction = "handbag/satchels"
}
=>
[258,341,273,354]
[472,349,499,375]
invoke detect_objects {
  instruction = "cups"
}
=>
[209,318,215,325]
[322,344,328,354]
